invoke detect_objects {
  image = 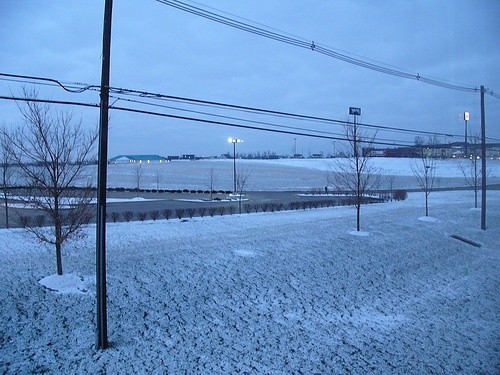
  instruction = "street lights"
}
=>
[226,136,244,195]
[463,111,470,158]
